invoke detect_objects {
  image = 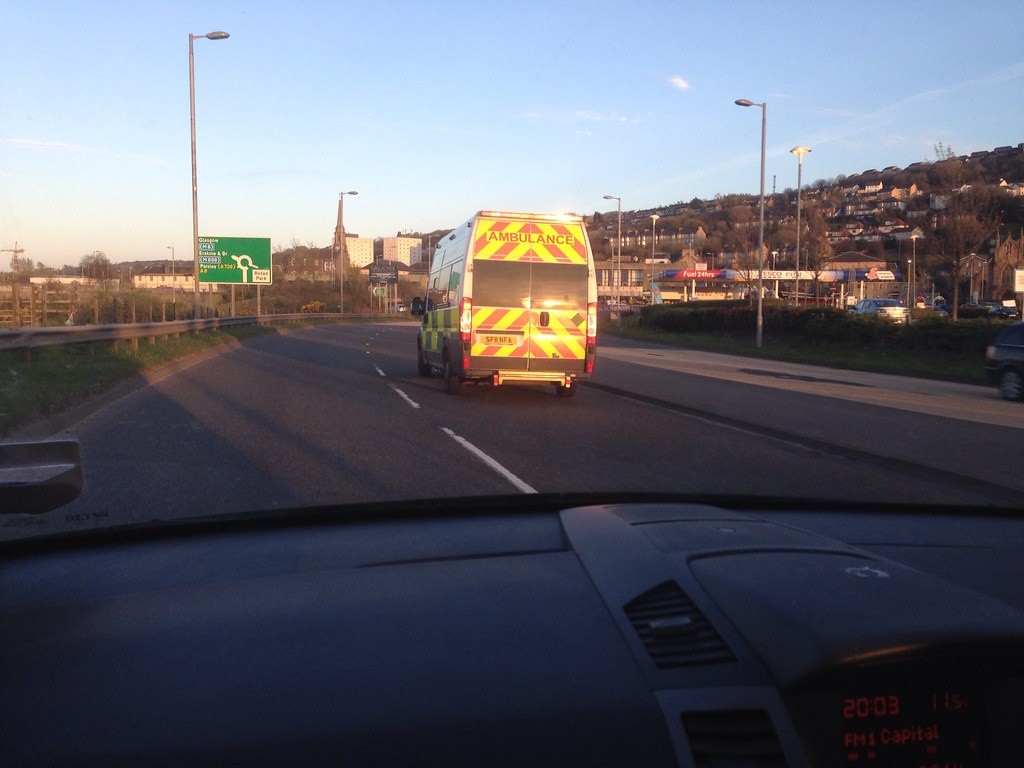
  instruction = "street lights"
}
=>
[649,214,661,304]
[603,195,622,329]
[910,234,919,309]
[340,190,359,313]
[789,146,812,305]
[167,246,175,303]
[428,234,440,274]
[188,30,230,322]
[735,99,767,348]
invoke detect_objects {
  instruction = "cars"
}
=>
[982,320,1024,399]
[848,298,913,327]
[923,296,1021,322]
[389,303,406,313]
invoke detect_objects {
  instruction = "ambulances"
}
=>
[410,209,598,399]
